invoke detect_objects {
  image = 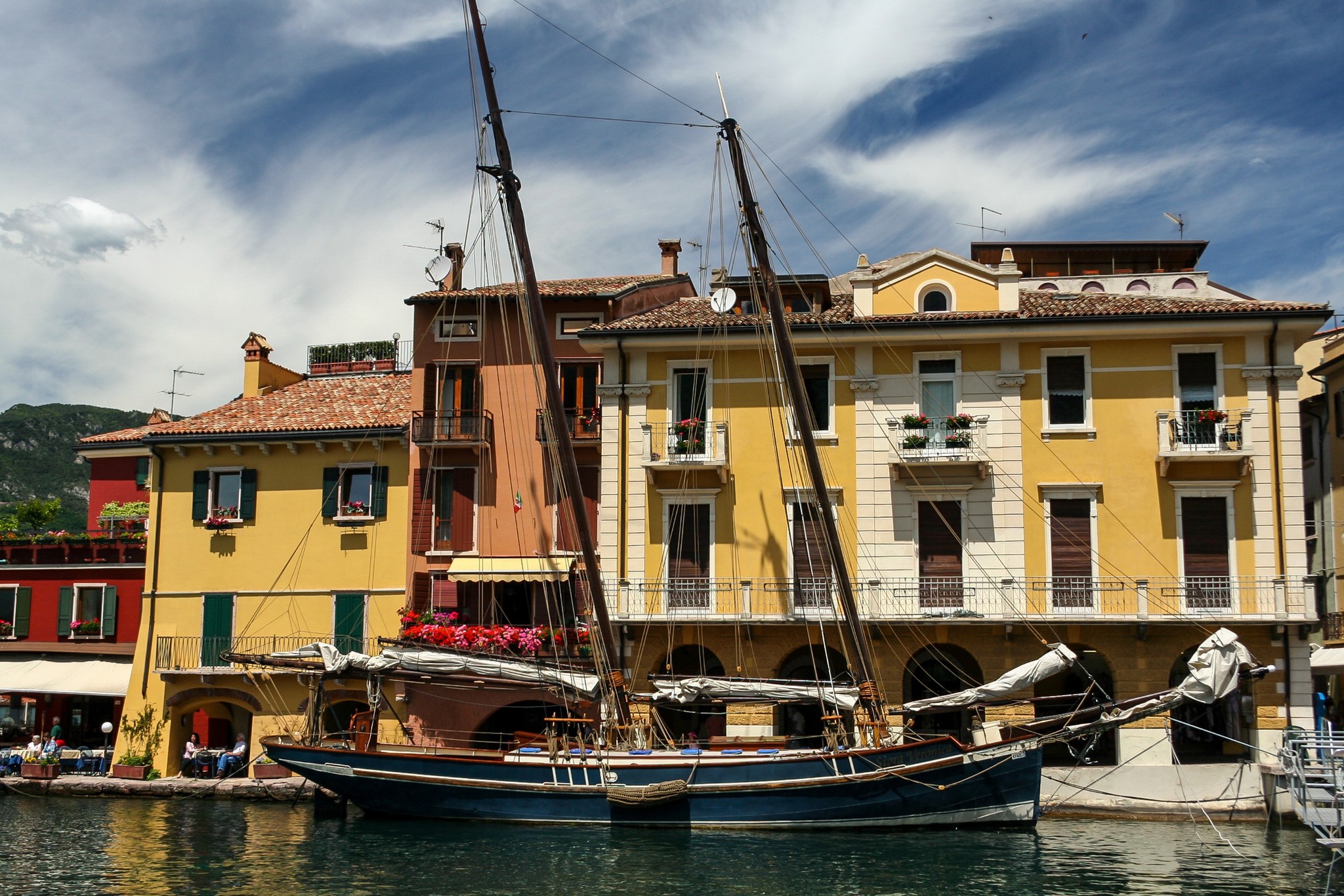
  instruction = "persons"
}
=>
[50,717,61,740]
[176,732,207,778]
[26,735,43,752]
[213,733,248,779]
[705,707,726,739]
[684,731,700,749]
[38,732,58,759]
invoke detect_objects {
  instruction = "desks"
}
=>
[192,749,232,776]
[1,749,115,773]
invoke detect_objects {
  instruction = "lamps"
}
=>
[101,722,113,733]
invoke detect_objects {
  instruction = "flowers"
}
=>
[579,407,597,425]
[0,620,12,631]
[0,531,148,550]
[25,755,59,766]
[206,506,237,526]
[902,414,971,447]
[254,758,273,763]
[397,605,591,656]
[1195,407,1229,424]
[70,617,100,631]
[346,502,366,513]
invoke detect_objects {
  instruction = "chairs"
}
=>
[1170,419,1199,445]
[1219,421,1242,449]
[79,748,94,775]
[181,749,250,781]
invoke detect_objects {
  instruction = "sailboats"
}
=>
[260,40,1281,830]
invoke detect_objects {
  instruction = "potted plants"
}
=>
[112,704,164,780]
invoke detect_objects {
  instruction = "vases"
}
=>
[91,538,116,544]
[904,423,928,429]
[217,513,236,519]
[252,764,292,779]
[120,538,141,544]
[0,627,12,635]
[205,523,233,530]
[65,539,90,544]
[345,511,367,516]
[947,422,970,431]
[21,763,60,780]
[37,539,57,545]
[946,442,968,449]
[7,541,31,545]
[74,629,100,636]
[903,442,926,450]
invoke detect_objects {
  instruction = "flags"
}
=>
[514,489,522,513]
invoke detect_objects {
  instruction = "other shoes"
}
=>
[214,775,223,779]
[176,774,180,777]
[217,771,224,775]
